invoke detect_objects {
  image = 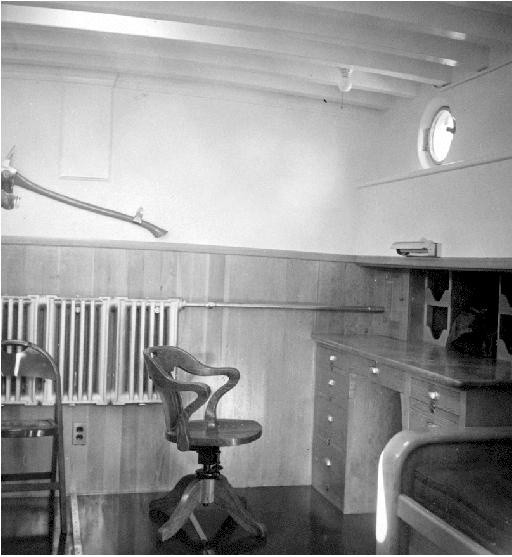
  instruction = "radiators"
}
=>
[1,295,182,407]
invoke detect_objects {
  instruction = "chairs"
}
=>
[143,346,270,542]
[375,426,512,555]
[1,340,67,533]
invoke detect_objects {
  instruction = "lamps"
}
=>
[339,68,353,93]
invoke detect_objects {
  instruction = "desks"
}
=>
[311,333,512,514]
